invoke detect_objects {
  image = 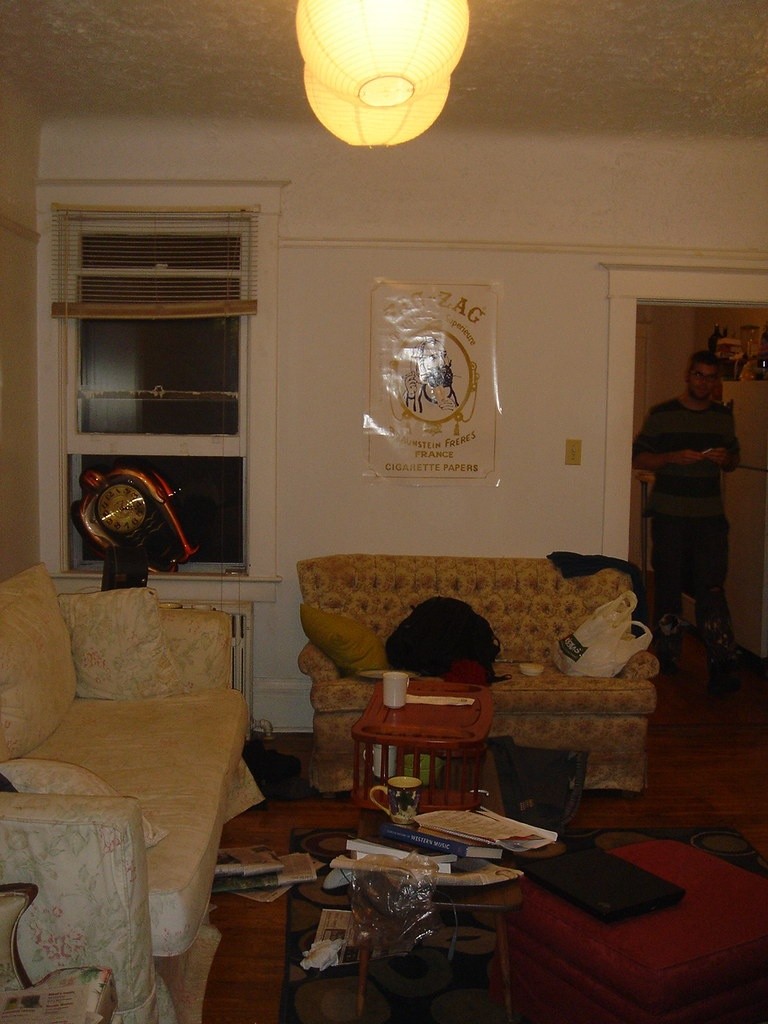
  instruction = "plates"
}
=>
[519,663,543,676]
[355,670,420,679]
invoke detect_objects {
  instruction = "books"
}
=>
[346,823,511,874]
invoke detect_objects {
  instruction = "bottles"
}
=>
[741,325,760,359]
[757,321,768,368]
[708,323,723,357]
[722,323,728,338]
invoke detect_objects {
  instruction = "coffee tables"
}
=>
[348,749,522,1024]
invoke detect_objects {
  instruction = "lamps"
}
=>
[295,0,470,146]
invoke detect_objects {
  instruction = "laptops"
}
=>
[518,847,685,921]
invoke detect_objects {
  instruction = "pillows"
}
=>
[299,603,390,672]
[0,759,169,850]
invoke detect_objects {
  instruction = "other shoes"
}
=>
[708,675,740,695]
[660,658,678,674]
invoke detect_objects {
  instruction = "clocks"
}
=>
[71,466,197,592]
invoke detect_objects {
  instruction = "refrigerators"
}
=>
[720,381,768,658]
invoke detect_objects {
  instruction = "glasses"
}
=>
[690,371,719,382]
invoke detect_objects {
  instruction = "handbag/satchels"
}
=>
[479,735,588,830]
[386,596,512,683]
[547,590,652,678]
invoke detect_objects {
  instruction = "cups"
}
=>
[369,776,422,825]
[363,744,396,779]
[382,672,410,710]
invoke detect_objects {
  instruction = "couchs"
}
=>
[295,554,659,796]
[0,562,249,1024]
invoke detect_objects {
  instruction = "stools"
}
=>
[490,839,768,1024]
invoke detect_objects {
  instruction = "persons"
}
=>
[633,350,743,699]
[752,332,768,381]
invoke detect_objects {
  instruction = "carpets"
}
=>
[279,827,768,1024]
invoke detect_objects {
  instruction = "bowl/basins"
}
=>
[402,753,442,786]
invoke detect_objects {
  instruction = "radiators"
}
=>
[158,598,273,741]
